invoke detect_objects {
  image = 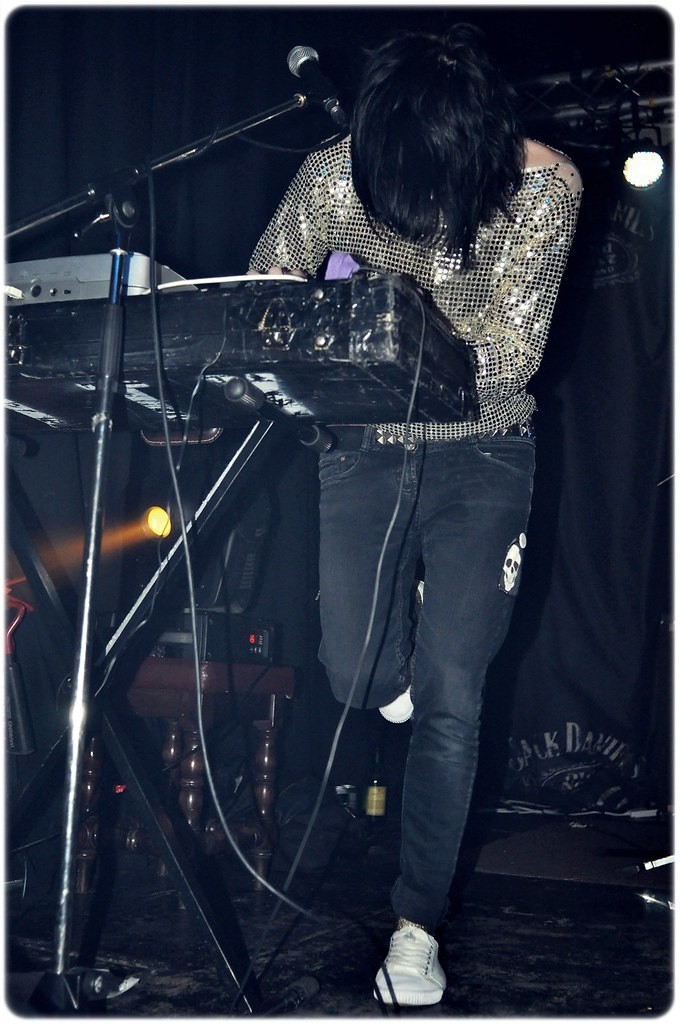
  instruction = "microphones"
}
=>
[287,46,350,132]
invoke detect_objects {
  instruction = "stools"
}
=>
[74,656,296,895]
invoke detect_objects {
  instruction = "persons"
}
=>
[245,29,584,1005]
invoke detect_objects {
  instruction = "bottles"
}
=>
[364,748,388,829]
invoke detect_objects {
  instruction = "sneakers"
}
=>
[378,581,424,723]
[373,926,446,1004]
